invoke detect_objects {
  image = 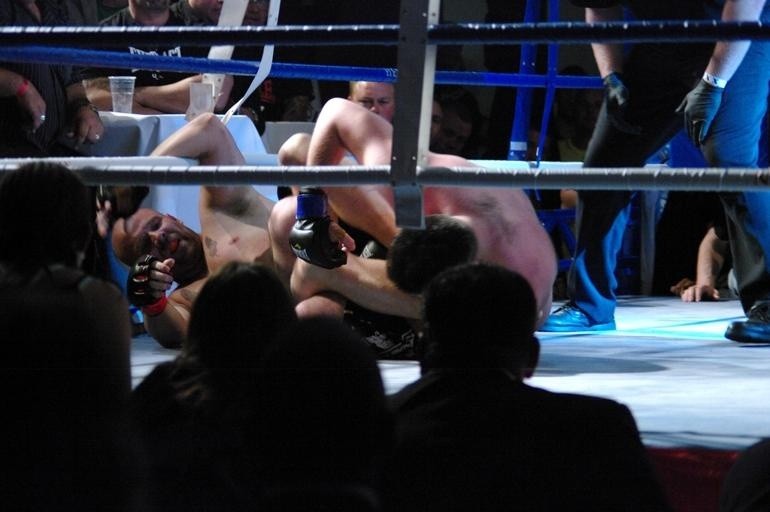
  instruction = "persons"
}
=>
[0,1,769,512]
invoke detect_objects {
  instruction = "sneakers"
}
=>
[538,302,615,332]
[725,307,770,344]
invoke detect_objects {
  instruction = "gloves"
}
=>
[603,72,643,136]
[95,184,149,226]
[675,71,727,148]
[126,255,172,317]
[288,187,347,269]
